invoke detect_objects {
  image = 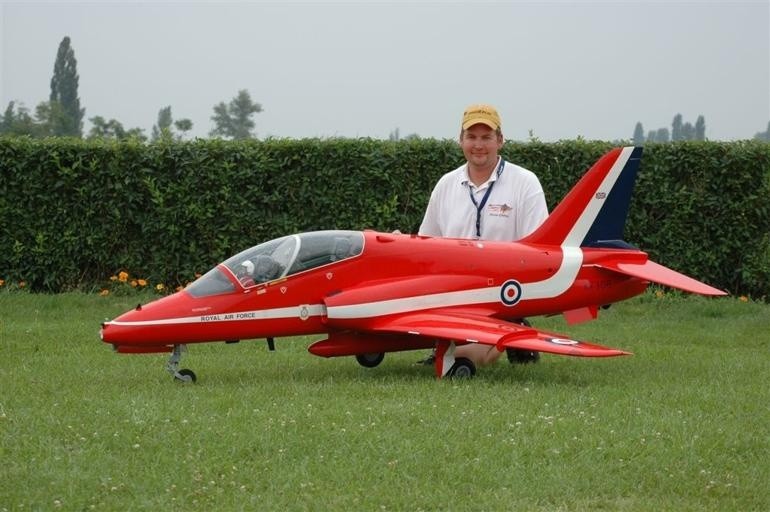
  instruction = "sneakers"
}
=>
[412,354,435,367]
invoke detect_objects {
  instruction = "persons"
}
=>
[239,260,255,287]
[417,103,549,364]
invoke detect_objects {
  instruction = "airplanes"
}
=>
[100,145,730,389]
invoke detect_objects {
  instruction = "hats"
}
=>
[462,103,501,130]
